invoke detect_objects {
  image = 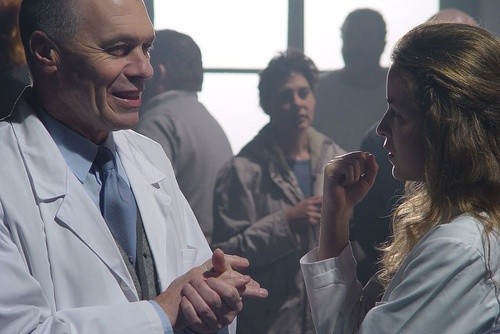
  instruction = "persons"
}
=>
[311,10,389,152]
[300,23,500,334]
[211,49,349,334]
[130,30,233,245]
[0,0,268,334]
[353,10,479,269]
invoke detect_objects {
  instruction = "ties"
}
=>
[93,146,138,270]
